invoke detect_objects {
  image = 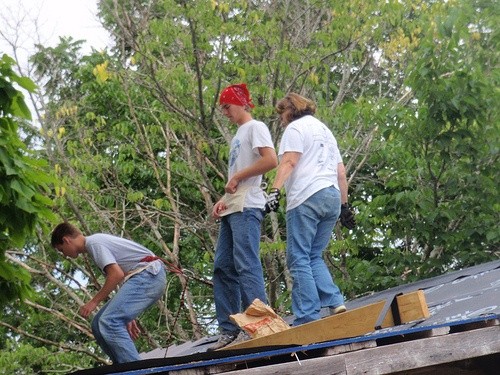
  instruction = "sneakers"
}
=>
[331,305,346,314]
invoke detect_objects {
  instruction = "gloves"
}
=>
[265,188,281,212]
[339,203,356,229]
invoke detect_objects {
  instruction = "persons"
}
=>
[51,223,166,363]
[206,83,277,352]
[266,92,347,327]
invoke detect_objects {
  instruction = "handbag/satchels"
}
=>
[229,298,291,340]
[226,330,252,349]
